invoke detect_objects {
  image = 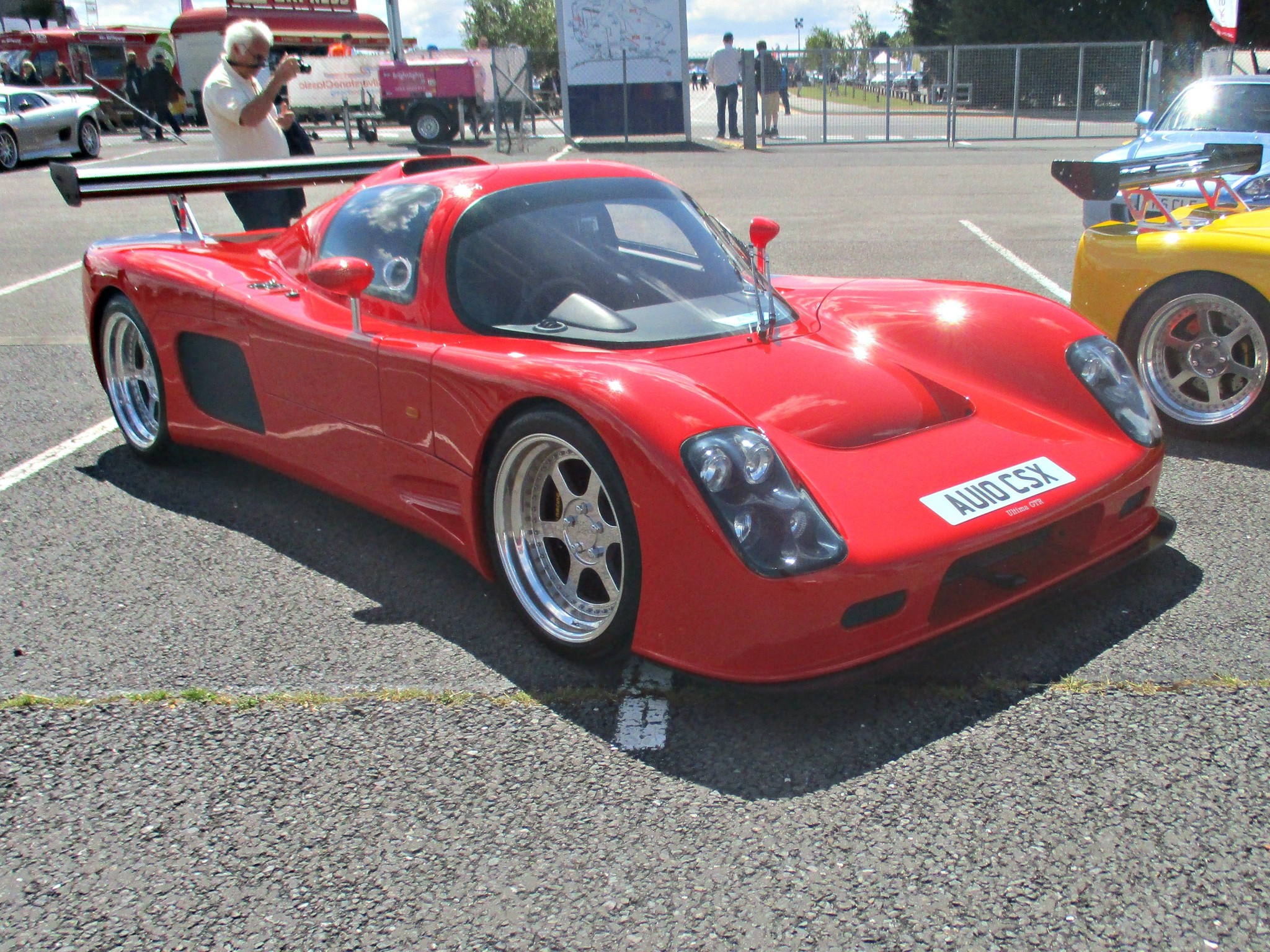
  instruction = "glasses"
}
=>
[241,44,269,64]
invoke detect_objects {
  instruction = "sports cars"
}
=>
[77,152,1171,683]
[0,88,104,169]
[1050,154,1269,445]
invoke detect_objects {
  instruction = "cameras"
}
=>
[295,59,310,73]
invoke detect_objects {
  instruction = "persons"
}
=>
[143,53,186,141]
[540,70,561,117]
[795,68,802,97]
[125,51,154,140]
[828,69,840,97]
[22,59,44,86]
[55,61,76,85]
[701,74,707,91]
[327,32,358,57]
[706,32,742,139]
[755,40,791,137]
[909,75,918,92]
[202,18,301,231]
[0,60,22,86]
[692,73,698,91]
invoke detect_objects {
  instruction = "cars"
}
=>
[871,71,923,90]
[1077,73,1270,238]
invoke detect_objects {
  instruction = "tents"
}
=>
[689,66,709,84]
[872,51,901,73]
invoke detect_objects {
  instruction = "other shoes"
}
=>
[770,127,778,135]
[758,129,772,138]
[730,133,744,139]
[717,134,724,138]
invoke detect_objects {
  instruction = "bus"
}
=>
[172,0,417,126]
[1,26,186,123]
